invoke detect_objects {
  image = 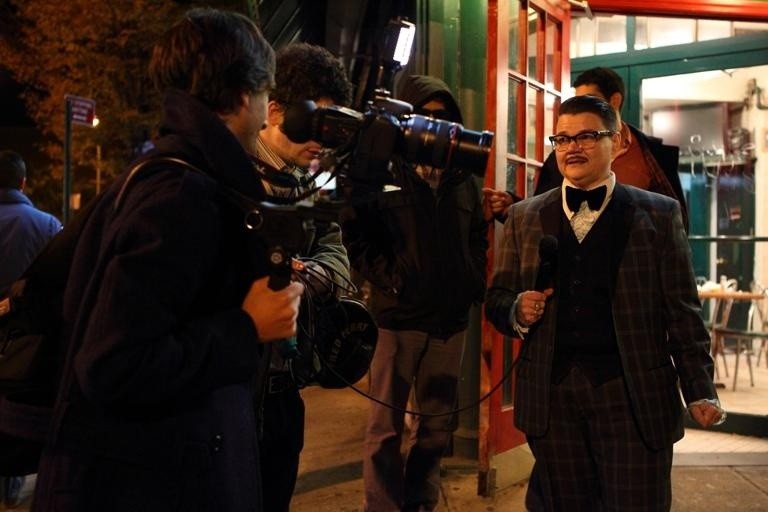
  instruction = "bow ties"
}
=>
[565,185,607,214]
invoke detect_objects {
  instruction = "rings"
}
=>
[534,301,541,311]
[534,310,538,317]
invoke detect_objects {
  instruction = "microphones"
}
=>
[531,234,556,334]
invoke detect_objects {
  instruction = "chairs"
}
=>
[710,279,759,392]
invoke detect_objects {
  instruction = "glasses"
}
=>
[549,131,612,152]
[415,106,447,120]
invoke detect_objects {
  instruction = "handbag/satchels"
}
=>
[309,295,379,390]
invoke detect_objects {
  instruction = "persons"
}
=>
[0,147,64,509]
[483,65,691,232]
[33,5,309,512]
[483,95,727,512]
[238,36,352,511]
[338,71,490,512]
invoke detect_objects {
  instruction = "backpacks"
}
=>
[0,155,204,479]
